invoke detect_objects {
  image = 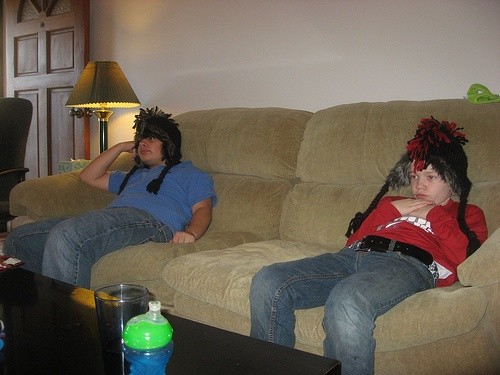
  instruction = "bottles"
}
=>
[121,301,175,375]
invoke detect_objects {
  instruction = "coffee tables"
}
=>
[0,266,342,375]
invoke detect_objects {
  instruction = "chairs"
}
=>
[0,97,34,230]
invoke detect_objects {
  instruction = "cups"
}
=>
[94,284,149,375]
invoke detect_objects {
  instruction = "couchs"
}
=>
[9,96,500,375]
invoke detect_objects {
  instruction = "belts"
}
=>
[348,235,439,285]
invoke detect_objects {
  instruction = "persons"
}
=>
[3,106,217,291]
[249,116,491,375]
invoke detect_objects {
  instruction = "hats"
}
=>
[345,115,482,258]
[115,106,182,193]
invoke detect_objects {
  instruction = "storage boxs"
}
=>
[57,160,92,175]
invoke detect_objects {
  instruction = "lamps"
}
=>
[65,60,141,153]
